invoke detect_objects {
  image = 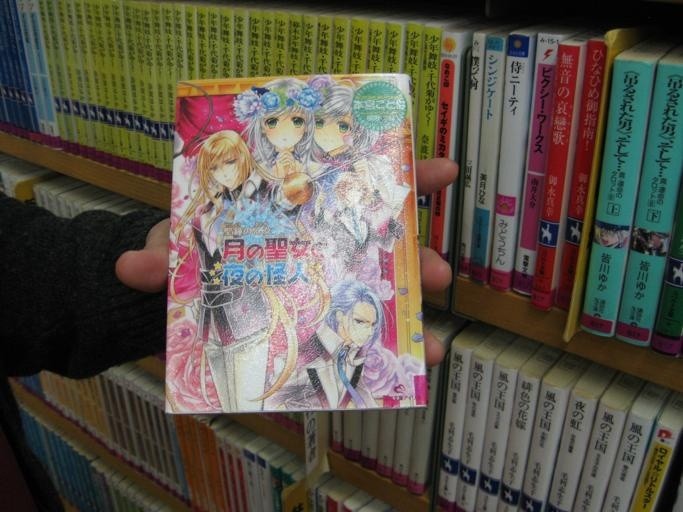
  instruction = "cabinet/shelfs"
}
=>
[0,0,683,512]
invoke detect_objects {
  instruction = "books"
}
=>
[407,23,683,358]
[167,414,429,511]
[430,316,683,512]
[165,73,429,414]
[12,359,166,511]
[177,2,406,80]
[0,1,173,176]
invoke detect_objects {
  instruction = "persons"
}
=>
[0,158,457,511]
